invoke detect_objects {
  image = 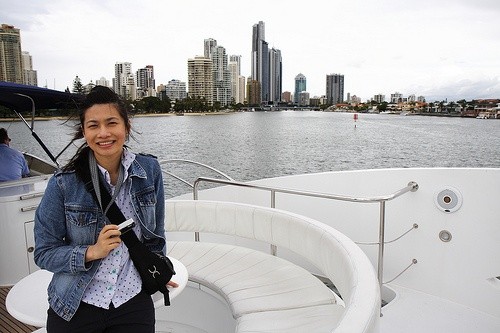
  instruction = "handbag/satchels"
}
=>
[128,238,174,294]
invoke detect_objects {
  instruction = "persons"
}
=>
[33,84,179,333]
[0,128,30,183]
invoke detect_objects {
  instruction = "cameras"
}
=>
[110,218,136,238]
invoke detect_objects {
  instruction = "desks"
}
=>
[4,254,189,333]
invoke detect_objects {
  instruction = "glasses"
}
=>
[8,138,11,141]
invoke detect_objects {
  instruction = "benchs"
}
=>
[164,199,382,333]
